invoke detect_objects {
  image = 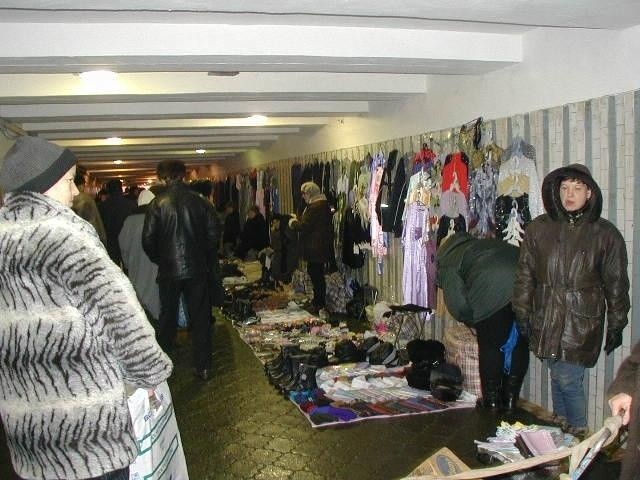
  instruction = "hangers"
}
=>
[290,120,547,246]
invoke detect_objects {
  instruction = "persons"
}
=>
[510,163,631,433]
[0,137,174,479]
[434,231,529,415]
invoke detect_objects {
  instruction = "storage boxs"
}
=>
[403,446,483,480]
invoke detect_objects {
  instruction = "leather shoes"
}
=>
[195,366,211,382]
[476,390,499,414]
[500,390,517,416]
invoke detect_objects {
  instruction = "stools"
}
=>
[388,302,434,362]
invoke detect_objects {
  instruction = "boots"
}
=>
[265,345,316,400]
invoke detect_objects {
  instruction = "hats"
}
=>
[250,205,259,214]
[137,189,156,206]
[0,134,79,197]
[300,181,321,195]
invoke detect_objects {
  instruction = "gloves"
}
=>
[603,330,623,356]
